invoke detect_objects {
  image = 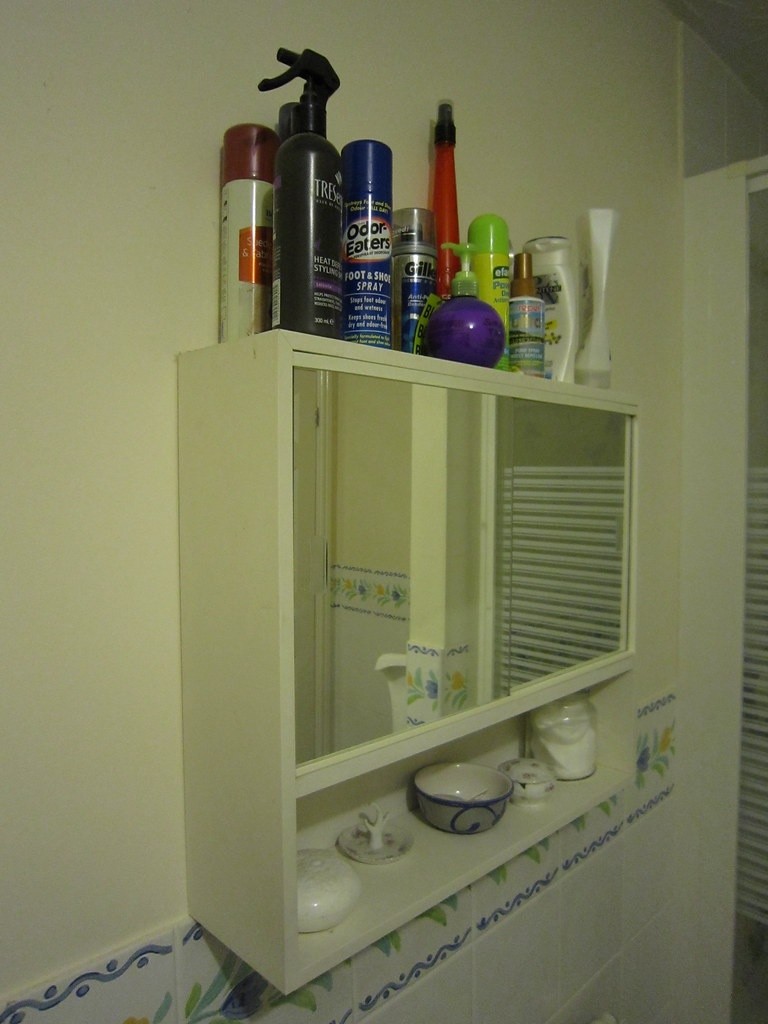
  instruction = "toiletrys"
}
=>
[216,50,620,386]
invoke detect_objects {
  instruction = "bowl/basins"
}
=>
[498,757,558,810]
[413,762,514,834]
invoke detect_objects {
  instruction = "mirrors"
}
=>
[294,369,631,763]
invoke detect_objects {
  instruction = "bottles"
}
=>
[529,691,599,781]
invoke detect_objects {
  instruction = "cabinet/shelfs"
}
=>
[176,327,642,994]
